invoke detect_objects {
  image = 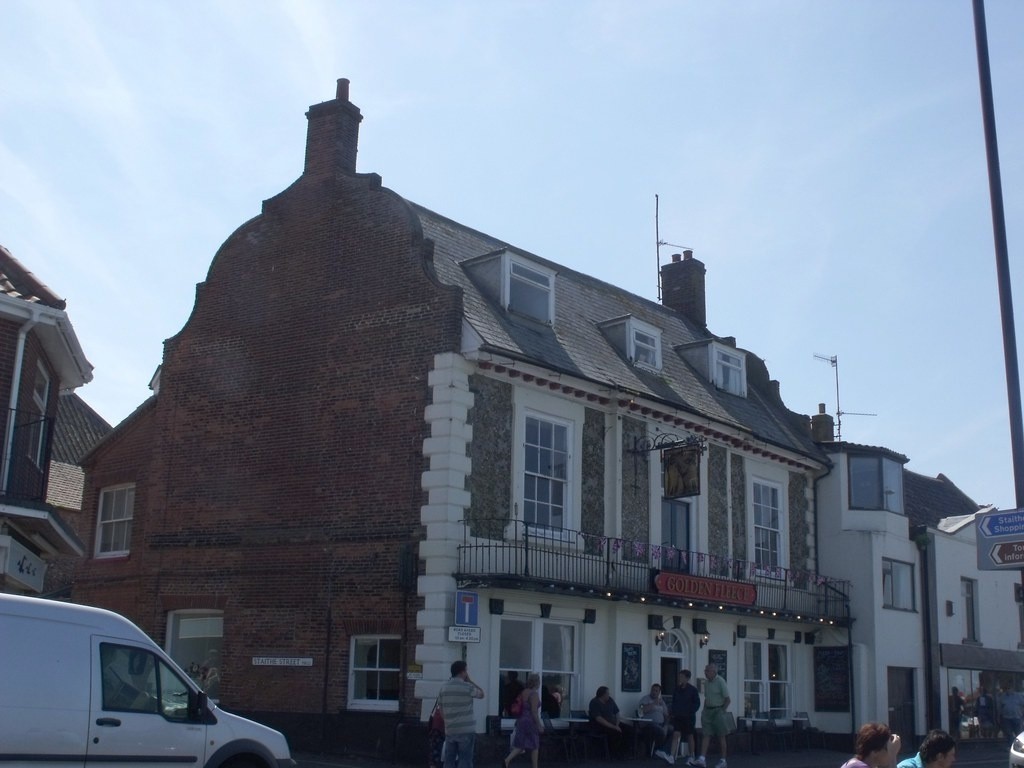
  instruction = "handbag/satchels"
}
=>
[510,696,523,716]
[427,715,433,732]
[510,727,525,754]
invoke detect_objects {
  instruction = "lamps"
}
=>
[700,630,711,649]
[655,623,666,646]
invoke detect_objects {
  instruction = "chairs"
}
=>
[486,710,827,765]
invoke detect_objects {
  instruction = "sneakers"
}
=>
[655,750,666,759]
[714,759,728,768]
[685,756,696,767]
[663,754,674,767]
[689,758,707,768]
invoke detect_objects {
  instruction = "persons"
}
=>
[639,684,669,760]
[999,687,1024,751]
[589,686,633,760]
[841,721,902,768]
[503,673,545,768]
[500,671,567,719]
[664,669,701,767]
[689,663,730,768]
[429,661,484,768]
[975,689,993,738]
[949,686,968,738]
[897,728,956,768]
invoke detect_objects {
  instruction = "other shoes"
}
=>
[503,759,511,768]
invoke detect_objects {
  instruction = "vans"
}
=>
[0,592,298,768]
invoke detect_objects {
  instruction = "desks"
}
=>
[737,717,769,756]
[554,718,589,764]
[625,718,653,761]
[785,718,809,752]
